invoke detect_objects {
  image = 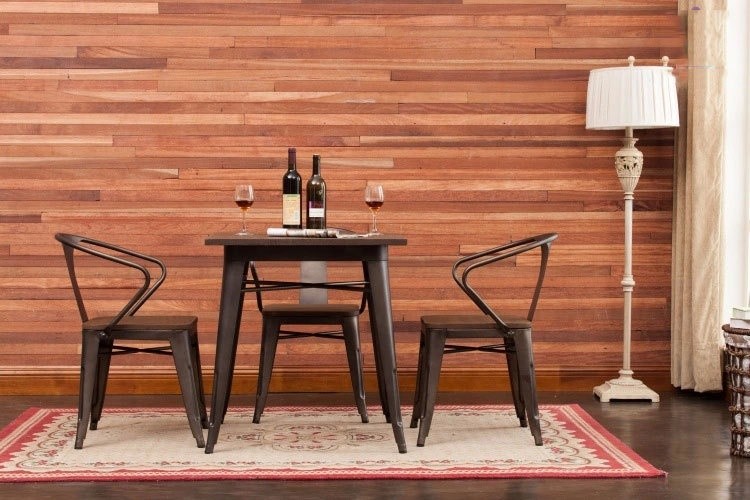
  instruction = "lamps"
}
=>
[586,56,680,403]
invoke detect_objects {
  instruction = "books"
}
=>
[267,228,369,238]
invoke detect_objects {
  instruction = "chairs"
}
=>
[55,232,209,449]
[249,227,369,424]
[410,233,558,447]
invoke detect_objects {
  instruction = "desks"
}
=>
[722,324,750,458]
[204,232,408,454]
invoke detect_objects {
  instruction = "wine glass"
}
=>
[364,184,384,236]
[234,184,254,236]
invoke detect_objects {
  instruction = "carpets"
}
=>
[0,404,668,483]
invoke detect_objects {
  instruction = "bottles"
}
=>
[306,154,326,229]
[282,148,302,229]
